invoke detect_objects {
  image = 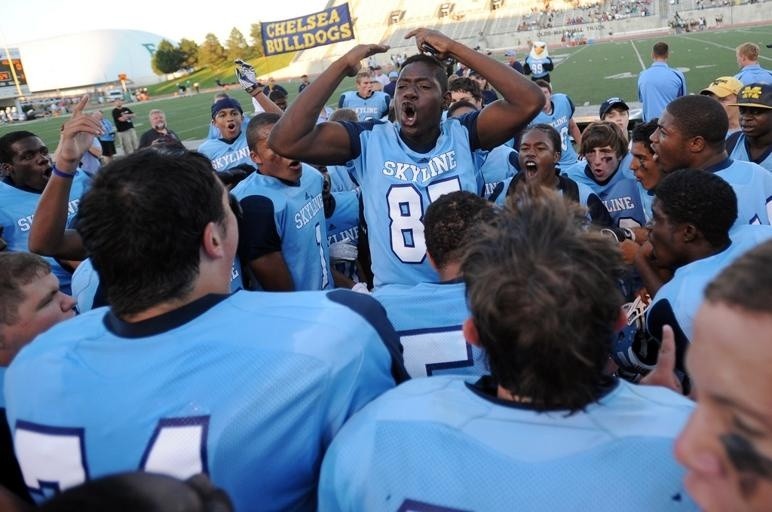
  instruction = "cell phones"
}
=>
[421,43,437,53]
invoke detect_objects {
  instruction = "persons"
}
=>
[672,234,770,511]
[263,27,546,285]
[320,178,703,511]
[27,466,237,512]
[0,2,770,397]
[2,151,409,512]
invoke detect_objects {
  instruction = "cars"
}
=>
[97,89,132,102]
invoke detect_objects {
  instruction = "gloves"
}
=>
[234,59,258,93]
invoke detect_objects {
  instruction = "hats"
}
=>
[600,97,629,118]
[699,76,771,110]
